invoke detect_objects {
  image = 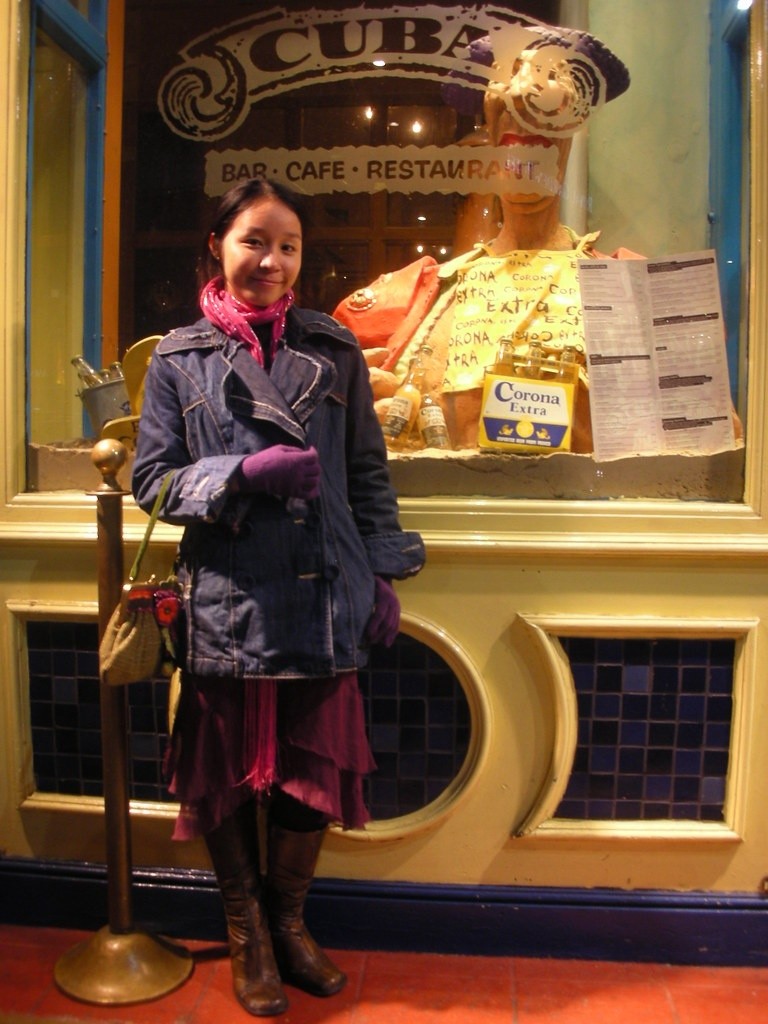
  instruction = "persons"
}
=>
[129,173,427,1016]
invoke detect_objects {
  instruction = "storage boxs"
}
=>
[476,370,575,458]
[27,436,138,495]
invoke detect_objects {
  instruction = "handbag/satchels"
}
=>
[98,469,184,686]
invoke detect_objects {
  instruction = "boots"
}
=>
[264,814,347,998]
[191,800,289,1016]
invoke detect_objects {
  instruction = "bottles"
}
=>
[71,355,125,386]
[421,393,451,451]
[559,348,576,383]
[493,338,515,375]
[381,378,421,452]
[526,341,541,378]
[407,346,433,387]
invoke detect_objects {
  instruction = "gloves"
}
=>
[364,574,400,651]
[235,443,322,501]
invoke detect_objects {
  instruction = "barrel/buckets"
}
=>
[76,378,131,439]
